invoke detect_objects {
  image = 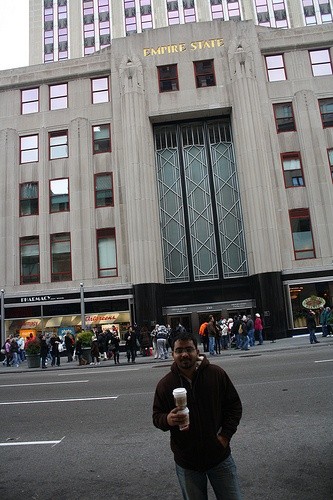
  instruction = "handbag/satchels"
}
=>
[1,350,6,354]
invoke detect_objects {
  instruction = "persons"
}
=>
[153,332,242,500]
[0,307,333,364]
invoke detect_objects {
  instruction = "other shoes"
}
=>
[42,365,47,368]
[115,361,121,364]
[154,354,162,358]
[51,363,62,367]
[310,340,320,344]
[322,334,326,337]
[127,359,130,363]
[241,348,250,351]
[217,352,222,354]
[132,360,136,363]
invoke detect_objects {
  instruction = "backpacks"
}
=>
[238,324,246,335]
[261,318,266,327]
[2,342,7,355]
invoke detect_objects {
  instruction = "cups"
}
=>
[177,407,189,430]
[172,388,187,406]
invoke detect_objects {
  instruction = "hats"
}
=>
[255,313,260,317]
